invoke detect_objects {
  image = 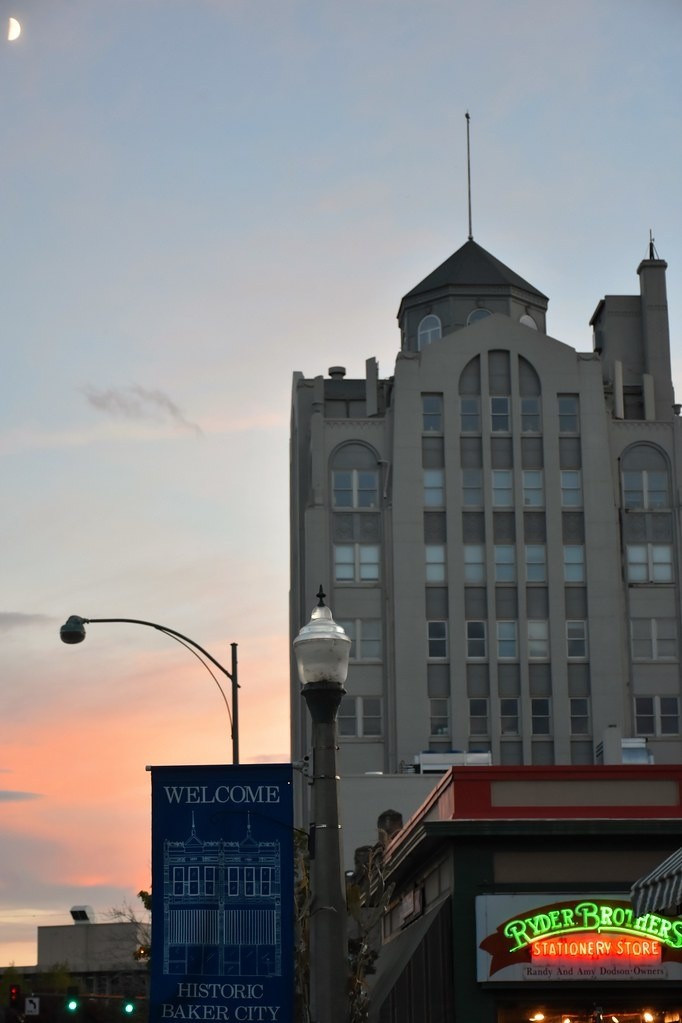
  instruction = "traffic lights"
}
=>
[9,984,21,1010]
[124,988,137,1017]
[67,986,80,1015]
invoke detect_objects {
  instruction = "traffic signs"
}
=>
[25,996,40,1015]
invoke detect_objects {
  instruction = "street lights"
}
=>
[61,612,239,765]
[291,582,354,1023]
